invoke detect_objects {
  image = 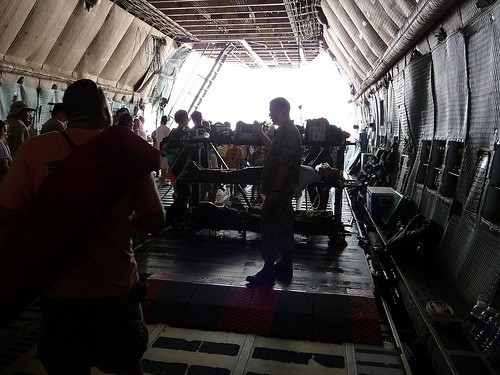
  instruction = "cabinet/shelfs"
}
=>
[182,138,347,251]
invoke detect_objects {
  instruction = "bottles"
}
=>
[464,301,500,356]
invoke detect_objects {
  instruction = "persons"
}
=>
[245,97,303,285]
[0,80,165,375]
[0,101,336,212]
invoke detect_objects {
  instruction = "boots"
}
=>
[246,264,275,284]
[274,258,293,283]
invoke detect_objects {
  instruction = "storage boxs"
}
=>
[366,187,394,221]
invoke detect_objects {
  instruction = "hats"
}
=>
[118,114,135,123]
[7,101,36,118]
